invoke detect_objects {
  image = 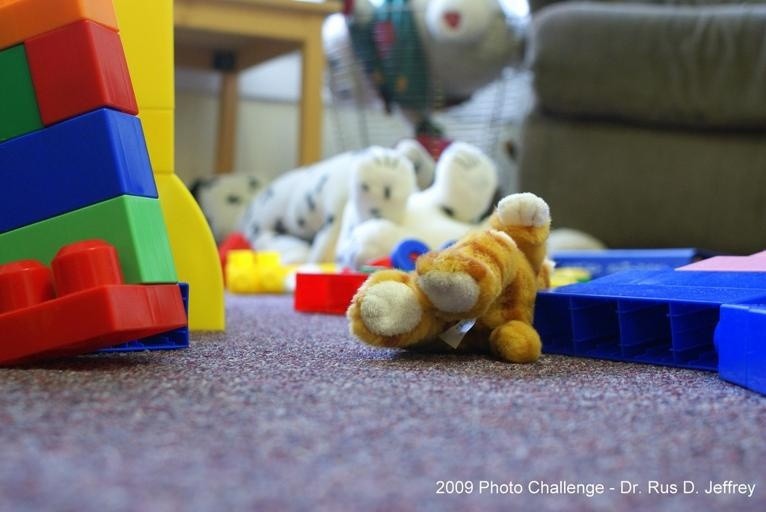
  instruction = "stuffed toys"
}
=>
[172,1,562,367]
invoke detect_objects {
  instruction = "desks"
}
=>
[174,0,343,176]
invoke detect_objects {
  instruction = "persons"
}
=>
[0,0,227,376]
[533,241,766,399]
